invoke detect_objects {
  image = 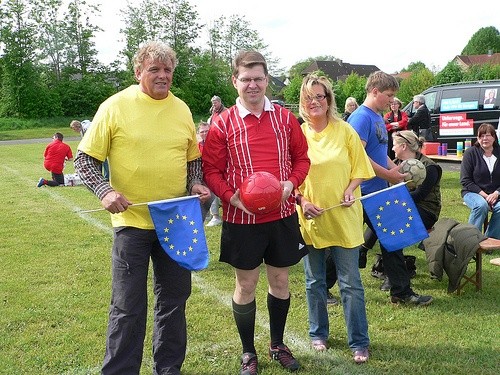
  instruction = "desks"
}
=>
[427,153,464,166]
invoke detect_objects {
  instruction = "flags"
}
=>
[360,185,430,252]
[145,195,210,272]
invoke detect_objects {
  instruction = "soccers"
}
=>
[401,158,426,187]
[238,172,282,214]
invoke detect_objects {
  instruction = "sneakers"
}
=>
[240,352,258,375]
[37,178,46,188]
[269,342,299,372]
[205,218,222,227]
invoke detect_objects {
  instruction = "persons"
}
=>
[459,124,500,240]
[70,119,110,184]
[341,94,443,290]
[197,95,229,227]
[289,70,376,363]
[485,90,497,104]
[37,132,74,187]
[203,50,311,375]
[75,41,211,375]
[347,72,434,306]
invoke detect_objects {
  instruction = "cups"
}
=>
[457,142,463,157]
[465,141,471,151]
[438,143,447,156]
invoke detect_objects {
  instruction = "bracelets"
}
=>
[295,194,303,206]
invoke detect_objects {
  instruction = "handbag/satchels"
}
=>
[371,253,416,279]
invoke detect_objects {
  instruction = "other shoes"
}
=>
[359,246,368,268]
[327,292,340,306]
[380,276,390,291]
[353,348,369,364]
[313,344,328,352]
[391,292,433,306]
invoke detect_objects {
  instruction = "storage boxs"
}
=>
[421,141,441,155]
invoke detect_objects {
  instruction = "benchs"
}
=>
[422,226,500,294]
[462,201,492,233]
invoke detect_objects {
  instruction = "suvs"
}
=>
[401,79,500,154]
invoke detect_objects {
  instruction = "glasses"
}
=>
[237,76,267,84]
[305,95,327,101]
[396,132,411,144]
